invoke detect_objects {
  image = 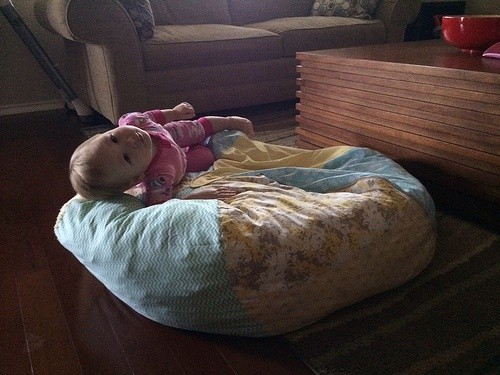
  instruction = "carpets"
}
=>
[282,209,500,375]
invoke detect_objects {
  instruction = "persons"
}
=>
[70,102,255,206]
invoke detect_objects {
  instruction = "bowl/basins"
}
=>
[441,13,500,54]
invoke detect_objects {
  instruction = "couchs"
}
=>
[33,0,421,126]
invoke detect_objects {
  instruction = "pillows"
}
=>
[309,0,379,20]
[119,0,155,40]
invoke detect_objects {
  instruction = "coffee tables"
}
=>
[295,38,500,234]
